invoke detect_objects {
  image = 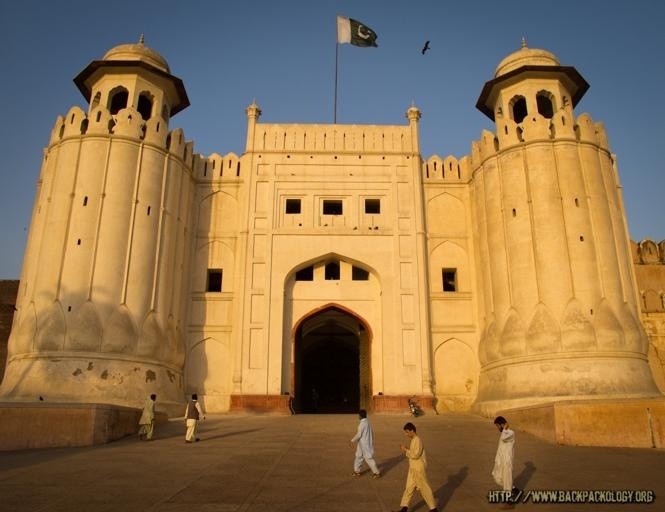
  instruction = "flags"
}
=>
[337,13,379,48]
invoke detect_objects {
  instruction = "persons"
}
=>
[137,393,158,440]
[397,422,440,512]
[348,409,382,480]
[184,393,204,443]
[312,387,319,413]
[491,415,520,510]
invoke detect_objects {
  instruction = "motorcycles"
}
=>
[408,398,420,418]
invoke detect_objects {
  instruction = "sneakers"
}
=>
[352,472,361,477]
[370,473,380,479]
